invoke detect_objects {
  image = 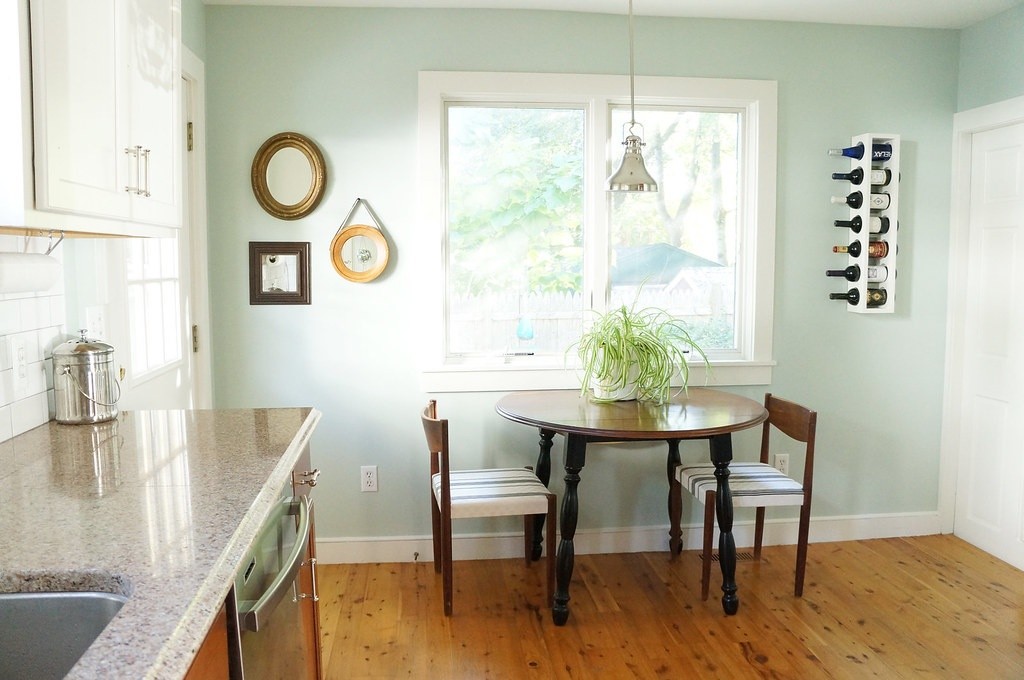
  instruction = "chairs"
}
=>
[420,398,558,620]
[671,392,817,602]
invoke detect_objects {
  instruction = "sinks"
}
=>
[0,570,133,680]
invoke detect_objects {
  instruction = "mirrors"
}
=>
[250,131,328,220]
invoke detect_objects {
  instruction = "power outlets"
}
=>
[360,466,378,493]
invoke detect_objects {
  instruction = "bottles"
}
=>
[833,241,898,258]
[834,215,900,234]
[682,336,690,361]
[831,191,891,210]
[829,288,896,306]
[832,169,901,187]
[825,265,898,283]
[827,144,892,161]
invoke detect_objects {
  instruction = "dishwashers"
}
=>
[224,468,311,679]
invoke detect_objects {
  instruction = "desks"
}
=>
[494,389,769,626]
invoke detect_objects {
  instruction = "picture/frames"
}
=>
[248,241,312,306]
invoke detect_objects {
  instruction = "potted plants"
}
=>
[564,305,716,409]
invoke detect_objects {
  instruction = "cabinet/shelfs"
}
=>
[2,1,182,240]
[291,444,323,679]
[185,599,229,680]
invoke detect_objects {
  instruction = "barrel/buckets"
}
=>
[51,327,121,425]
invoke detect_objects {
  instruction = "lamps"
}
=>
[603,0,658,192]
